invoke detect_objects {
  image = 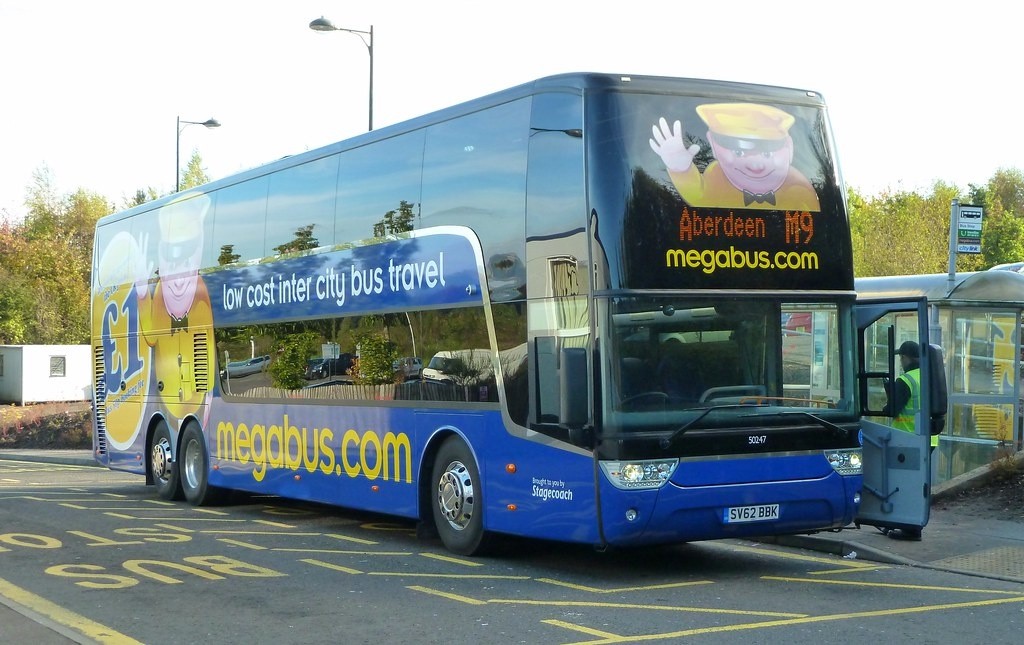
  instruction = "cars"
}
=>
[301,350,357,381]
[780,310,815,335]
[390,356,424,384]
[227,354,271,378]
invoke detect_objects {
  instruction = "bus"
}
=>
[91,70,948,557]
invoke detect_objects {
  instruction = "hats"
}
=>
[893,341,919,358]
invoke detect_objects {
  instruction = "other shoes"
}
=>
[874,526,889,534]
[887,529,921,541]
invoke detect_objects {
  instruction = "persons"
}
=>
[884,341,940,541]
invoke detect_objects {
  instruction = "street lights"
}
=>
[311,14,374,133]
[175,115,222,194]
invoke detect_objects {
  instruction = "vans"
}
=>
[421,349,495,383]
